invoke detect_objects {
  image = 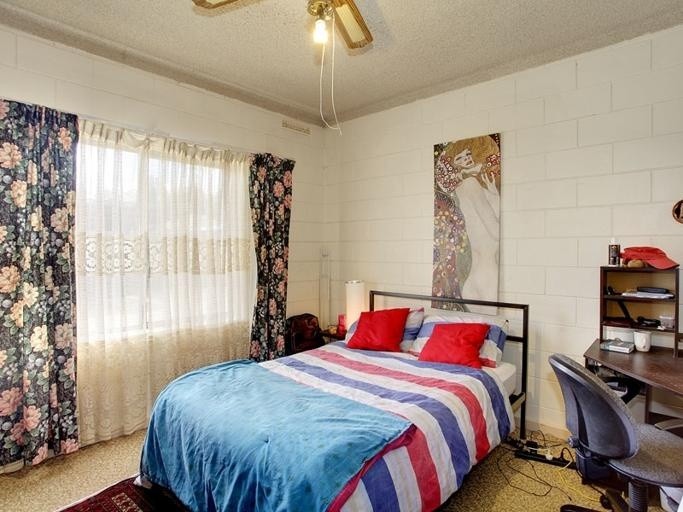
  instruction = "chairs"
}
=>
[284,314,342,356]
[549,353,682,512]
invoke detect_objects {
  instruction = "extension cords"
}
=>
[514,449,578,470]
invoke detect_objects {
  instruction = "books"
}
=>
[600,340,633,354]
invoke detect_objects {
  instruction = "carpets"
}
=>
[60,476,182,512]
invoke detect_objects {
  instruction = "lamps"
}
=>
[308,1,333,45]
[344,279,366,333]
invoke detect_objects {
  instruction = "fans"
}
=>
[189,0,374,49]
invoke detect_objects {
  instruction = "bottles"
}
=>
[607,238,620,269]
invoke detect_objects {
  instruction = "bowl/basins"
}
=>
[327,325,337,334]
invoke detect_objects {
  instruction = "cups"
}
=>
[631,330,651,352]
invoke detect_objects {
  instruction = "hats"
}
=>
[619,246,680,270]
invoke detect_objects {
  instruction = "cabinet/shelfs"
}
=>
[598,266,680,354]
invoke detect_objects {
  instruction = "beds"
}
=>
[135,290,528,512]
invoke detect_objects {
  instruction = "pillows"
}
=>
[419,323,492,369]
[345,306,424,355]
[349,308,410,353]
[410,317,509,369]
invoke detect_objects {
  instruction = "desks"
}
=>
[322,326,344,346]
[577,338,682,501]
